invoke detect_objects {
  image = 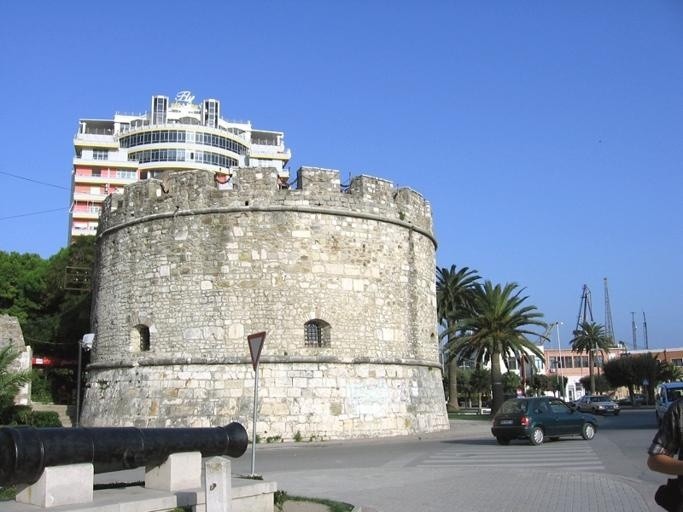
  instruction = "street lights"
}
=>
[556,320,565,401]
[77,333,95,427]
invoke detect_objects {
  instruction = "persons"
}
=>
[647,394,683,512]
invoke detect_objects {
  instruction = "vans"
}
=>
[656,382,683,426]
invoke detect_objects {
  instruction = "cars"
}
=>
[491,395,620,446]
